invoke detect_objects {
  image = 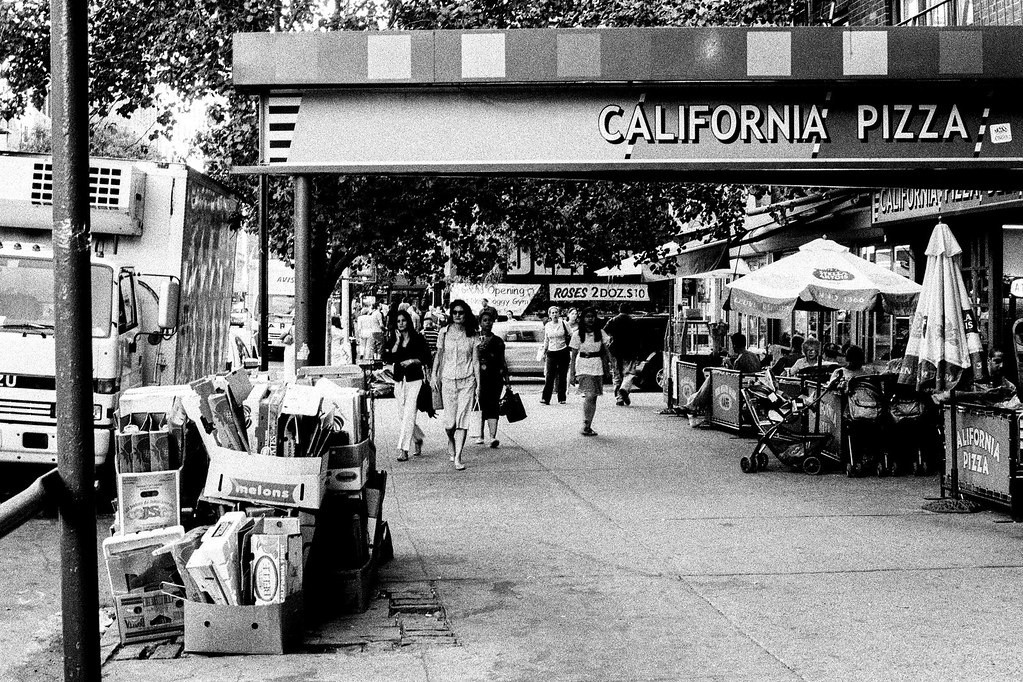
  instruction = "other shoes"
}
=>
[489,439,499,448]
[619,388,631,405]
[559,401,566,404]
[476,437,484,444]
[455,461,464,469]
[449,452,455,462]
[397,453,409,461]
[579,427,598,435]
[540,399,550,405]
[616,396,624,406]
[413,439,422,455]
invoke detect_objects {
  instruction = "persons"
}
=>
[477,309,510,449]
[430,298,480,471]
[603,300,640,408]
[568,306,609,435]
[729,331,868,387]
[328,297,451,366]
[383,309,434,461]
[476,297,518,322]
[537,305,579,406]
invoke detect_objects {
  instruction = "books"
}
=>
[185,366,370,459]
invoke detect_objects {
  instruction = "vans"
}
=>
[232,289,250,328]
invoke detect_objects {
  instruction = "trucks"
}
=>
[245,244,300,361]
[0,152,245,494]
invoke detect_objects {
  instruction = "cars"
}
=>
[496,320,550,377]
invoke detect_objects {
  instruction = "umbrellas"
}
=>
[719,236,922,434]
[897,221,984,501]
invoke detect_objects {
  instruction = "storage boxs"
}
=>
[106,363,395,661]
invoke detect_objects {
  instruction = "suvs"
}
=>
[629,311,669,389]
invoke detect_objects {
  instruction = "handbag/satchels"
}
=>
[503,387,527,423]
[562,320,573,351]
[417,380,436,418]
[499,388,513,416]
[599,328,610,364]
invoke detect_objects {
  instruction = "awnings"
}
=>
[592,193,849,283]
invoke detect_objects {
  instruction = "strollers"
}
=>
[735,369,843,479]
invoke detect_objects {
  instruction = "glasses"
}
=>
[806,349,817,353]
